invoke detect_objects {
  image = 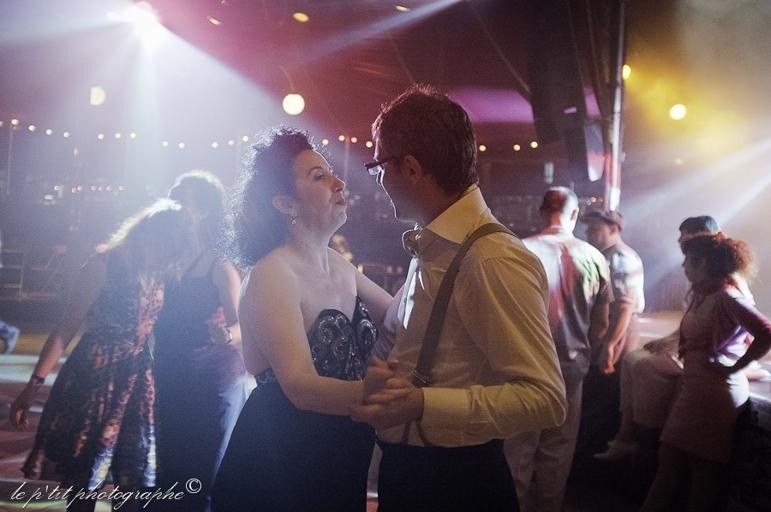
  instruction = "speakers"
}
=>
[524,0,603,144]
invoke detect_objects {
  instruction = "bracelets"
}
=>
[31,375,45,383]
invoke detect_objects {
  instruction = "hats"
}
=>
[579,206,623,231]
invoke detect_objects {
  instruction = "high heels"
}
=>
[592,444,642,467]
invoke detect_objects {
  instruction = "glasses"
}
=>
[363,162,393,176]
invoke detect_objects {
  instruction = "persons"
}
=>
[10,200,200,512]
[640,234,771,512]
[0,320,19,354]
[152,170,247,512]
[210,125,394,512]
[353,83,570,512]
[593,216,756,468]
[502,186,615,512]
[571,210,646,474]
